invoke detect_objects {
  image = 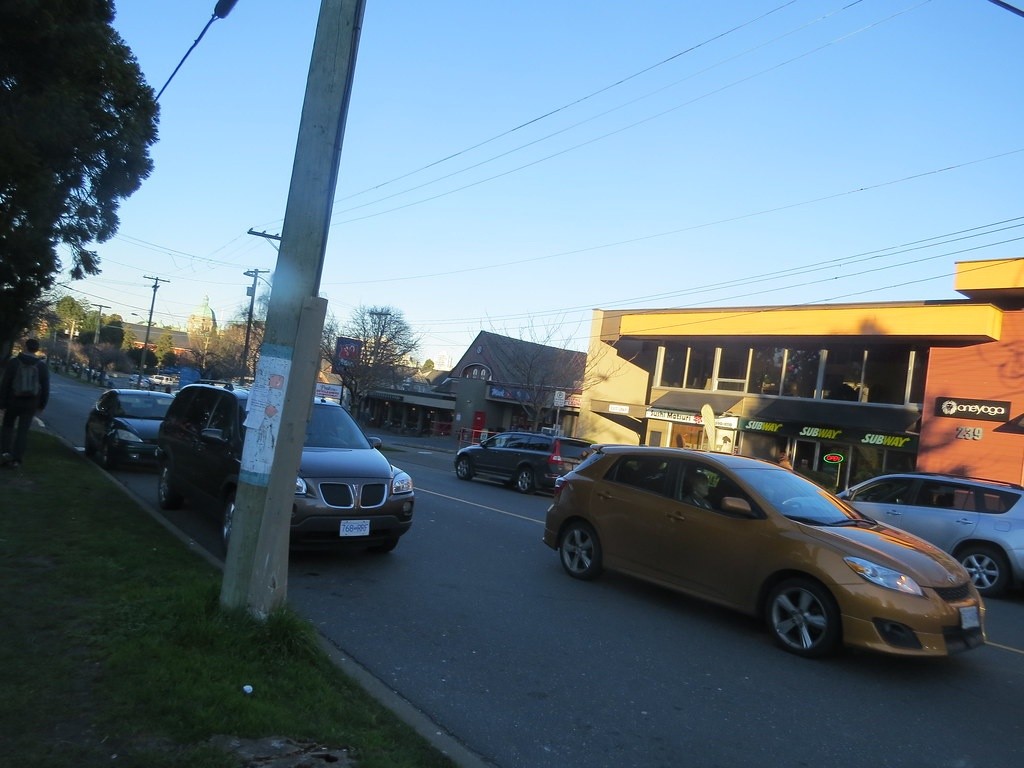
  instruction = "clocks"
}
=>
[476,345,483,354]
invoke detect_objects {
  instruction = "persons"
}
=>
[150,382,155,390]
[0,339,50,466]
[165,385,171,394]
[796,457,811,471]
[779,450,794,470]
[682,472,713,509]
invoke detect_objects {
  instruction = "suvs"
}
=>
[153,383,415,551]
[782,471,1024,596]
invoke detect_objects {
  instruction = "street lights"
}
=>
[131,312,153,388]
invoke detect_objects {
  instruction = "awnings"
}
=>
[647,391,744,416]
[757,399,922,435]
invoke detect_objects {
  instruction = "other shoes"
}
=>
[2,452,13,464]
[8,460,21,468]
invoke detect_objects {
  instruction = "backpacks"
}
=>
[10,357,44,400]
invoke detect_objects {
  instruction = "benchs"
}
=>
[184,397,225,422]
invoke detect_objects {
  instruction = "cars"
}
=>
[543,444,988,659]
[130,374,174,389]
[86,389,174,469]
[453,430,597,495]
[73,362,110,382]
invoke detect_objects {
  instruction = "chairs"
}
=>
[620,464,641,483]
[927,494,946,505]
[717,477,736,497]
[120,402,134,416]
[888,485,910,503]
[151,400,170,417]
[311,411,339,445]
